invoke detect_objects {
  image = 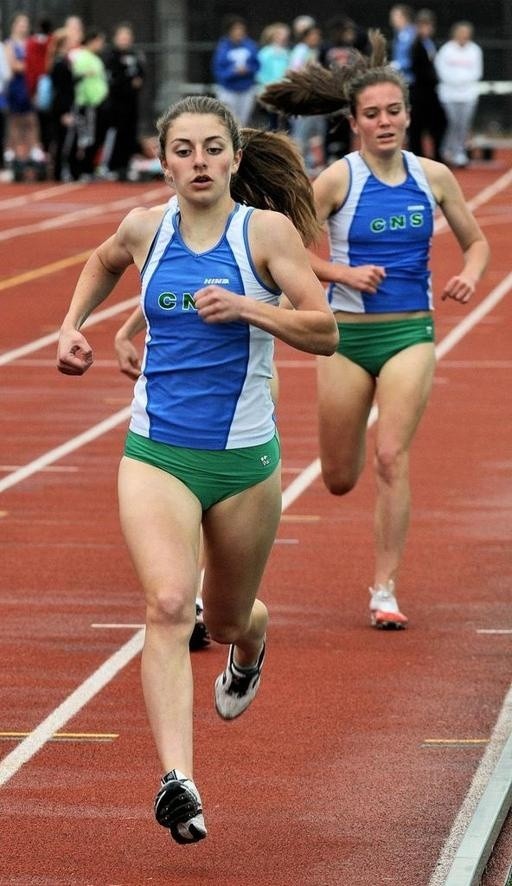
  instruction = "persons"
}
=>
[213,5,484,170]
[0,12,163,183]
[259,29,491,631]
[57,96,341,845]
[113,128,319,653]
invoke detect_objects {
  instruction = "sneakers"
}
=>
[368,581,408,628]
[215,640,265,720]
[154,769,208,846]
